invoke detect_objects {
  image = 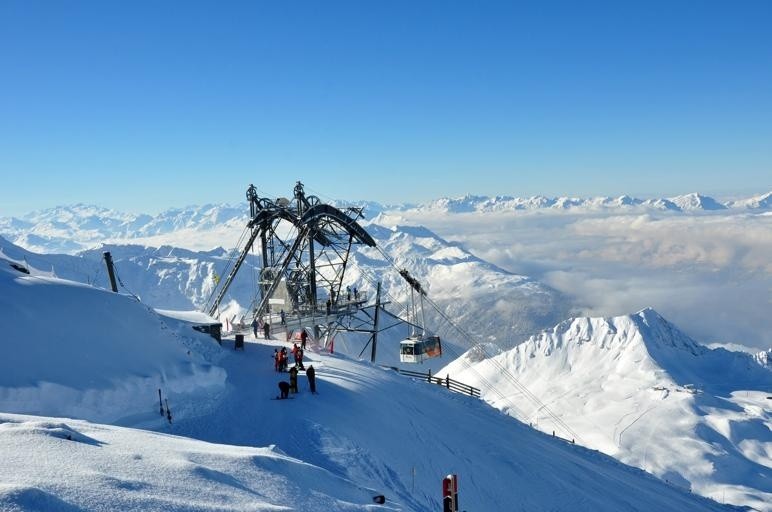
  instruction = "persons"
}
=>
[277,381,291,399]
[248,283,365,373]
[288,366,300,394]
[305,364,317,393]
